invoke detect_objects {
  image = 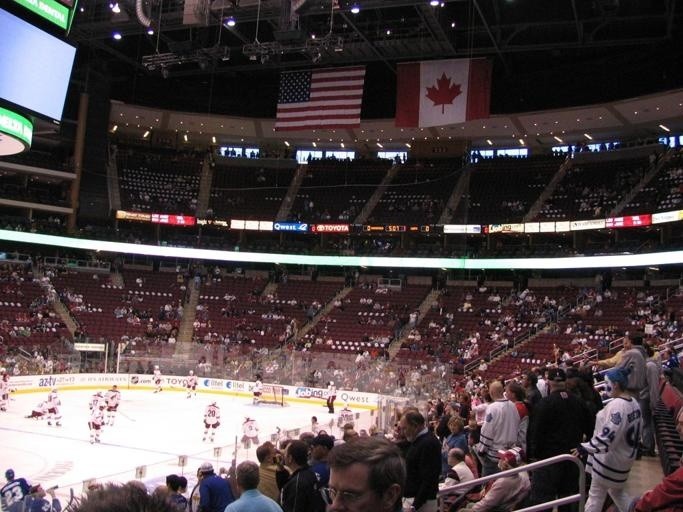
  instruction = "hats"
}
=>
[5,469,14,478]
[547,368,567,383]
[497,446,525,467]
[199,462,214,473]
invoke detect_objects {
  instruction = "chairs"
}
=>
[0,136,682,395]
[644,380,681,479]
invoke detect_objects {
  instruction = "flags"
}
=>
[273,66,367,131]
[394,58,494,129]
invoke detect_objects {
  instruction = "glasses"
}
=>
[319,487,384,508]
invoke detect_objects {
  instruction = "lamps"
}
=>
[148,33,342,79]
